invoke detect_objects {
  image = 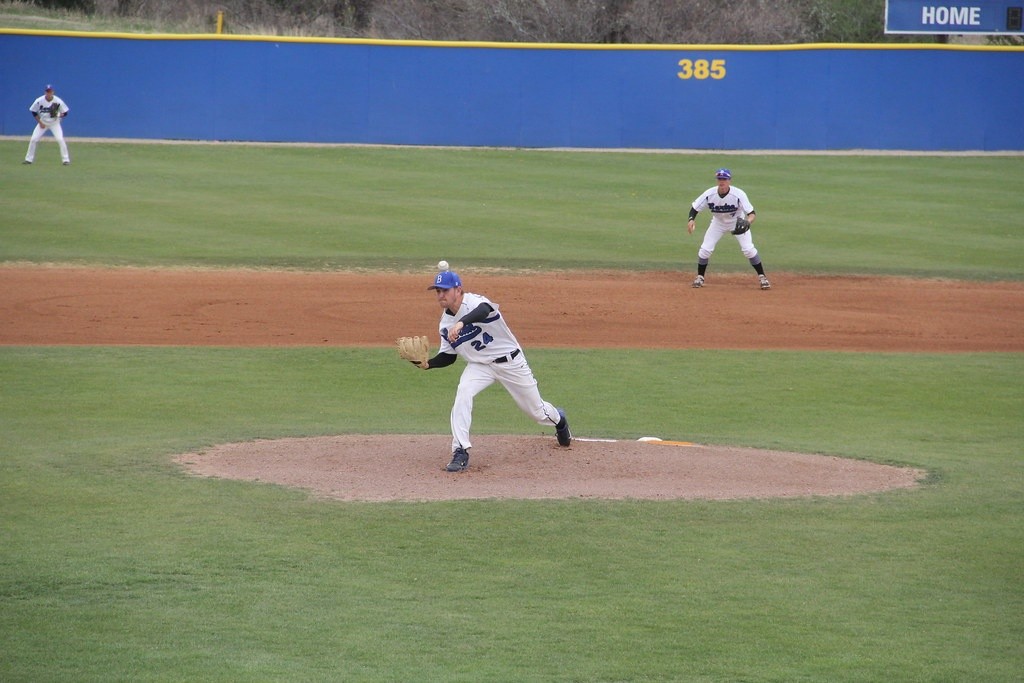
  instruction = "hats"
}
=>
[426,271,461,289]
[43,84,54,91]
[714,168,731,180]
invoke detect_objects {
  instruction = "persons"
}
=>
[687,165,772,291]
[24,82,70,167]
[393,270,572,473]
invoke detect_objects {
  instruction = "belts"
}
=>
[495,349,520,364]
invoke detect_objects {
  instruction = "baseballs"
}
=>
[438,260,448,271]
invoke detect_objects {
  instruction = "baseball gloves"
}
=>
[50,103,57,117]
[395,336,429,370]
[730,216,750,235]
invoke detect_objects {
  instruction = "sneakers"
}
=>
[758,274,770,289]
[22,160,33,164]
[691,275,704,288]
[446,446,469,472]
[556,409,572,447]
[62,161,70,165]
